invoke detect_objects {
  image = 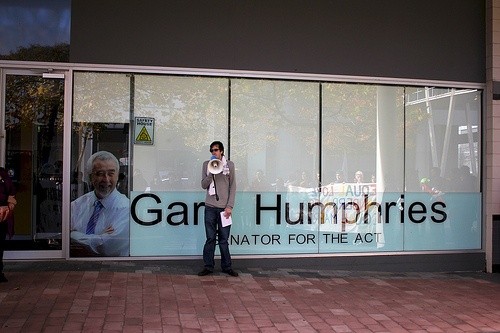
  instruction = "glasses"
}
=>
[210,149,220,152]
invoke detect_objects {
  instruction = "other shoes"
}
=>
[198,268,214,275]
[49,239,58,244]
[222,268,238,276]
[0,272,8,282]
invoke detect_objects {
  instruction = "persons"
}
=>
[70,150,130,256]
[198,141,238,277]
[0,167,17,284]
[252,166,478,246]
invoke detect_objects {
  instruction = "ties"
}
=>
[86,200,104,235]
